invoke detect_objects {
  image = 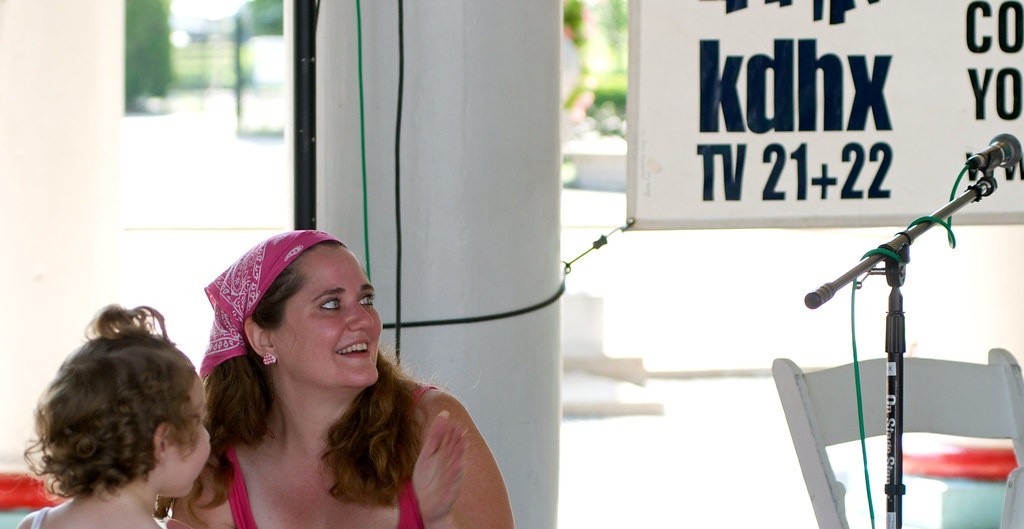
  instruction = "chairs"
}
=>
[772,349,1024,529]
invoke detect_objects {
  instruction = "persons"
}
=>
[2,305,211,529]
[155,229,515,529]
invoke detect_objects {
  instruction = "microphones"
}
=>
[964,133,1021,170]
